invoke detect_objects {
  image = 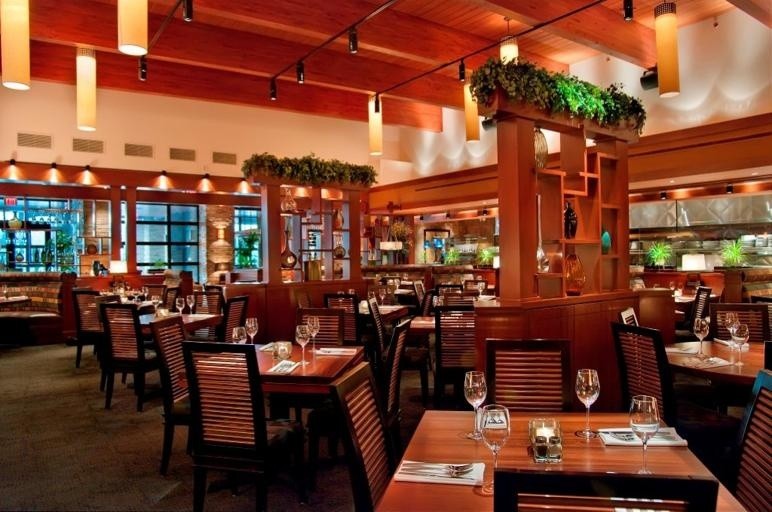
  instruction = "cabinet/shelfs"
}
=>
[500,113,630,411]
[261,174,360,338]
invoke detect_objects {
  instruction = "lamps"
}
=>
[118,2,148,57]
[464,82,480,143]
[500,37,518,65]
[0,2,29,90]
[654,4,680,100]
[369,98,384,154]
[77,49,97,132]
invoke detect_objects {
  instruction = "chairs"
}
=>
[492,467,718,511]
[329,362,395,512]
[433,305,489,404]
[192,291,222,314]
[98,303,158,414]
[708,300,770,340]
[485,337,572,411]
[462,279,487,294]
[324,293,360,344]
[441,291,480,307]
[149,317,186,477]
[368,297,428,409]
[295,306,343,425]
[163,286,182,309]
[215,294,249,342]
[436,285,463,297]
[728,368,772,511]
[180,340,311,512]
[412,280,432,314]
[373,319,411,443]
[610,321,713,426]
[70,287,101,369]
[203,285,225,337]
[143,285,167,304]
[691,286,712,324]
[618,307,727,415]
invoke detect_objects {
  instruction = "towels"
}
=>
[269,360,300,375]
[188,313,215,318]
[598,426,688,447]
[316,347,356,355]
[259,342,275,352]
[714,337,733,346]
[393,459,485,487]
[665,346,699,354]
[707,356,729,367]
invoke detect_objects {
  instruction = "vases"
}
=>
[333,243,346,258]
[280,186,298,213]
[534,126,548,168]
[8,210,22,229]
[16,251,24,262]
[281,231,297,268]
[333,209,344,228]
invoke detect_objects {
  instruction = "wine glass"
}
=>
[337,287,386,310]
[731,323,749,367]
[463,371,487,440]
[244,317,258,344]
[296,325,310,366]
[627,395,660,475]
[307,316,320,352]
[724,311,737,348]
[375,271,409,289]
[175,297,183,314]
[231,327,247,343]
[574,368,598,439]
[187,295,194,317]
[476,403,509,494]
[100,286,150,302]
[151,296,159,313]
[478,281,485,297]
[693,317,710,356]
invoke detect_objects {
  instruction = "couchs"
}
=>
[0,272,77,339]
